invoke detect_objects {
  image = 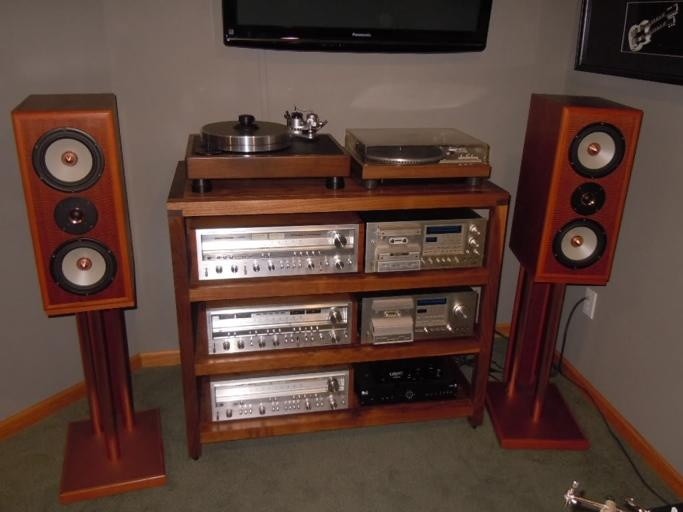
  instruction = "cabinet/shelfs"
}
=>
[165,156,512,463]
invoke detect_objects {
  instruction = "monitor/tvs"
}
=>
[222,0,493,54]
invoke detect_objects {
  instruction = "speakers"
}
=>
[11,93,136,319]
[510,93,644,286]
